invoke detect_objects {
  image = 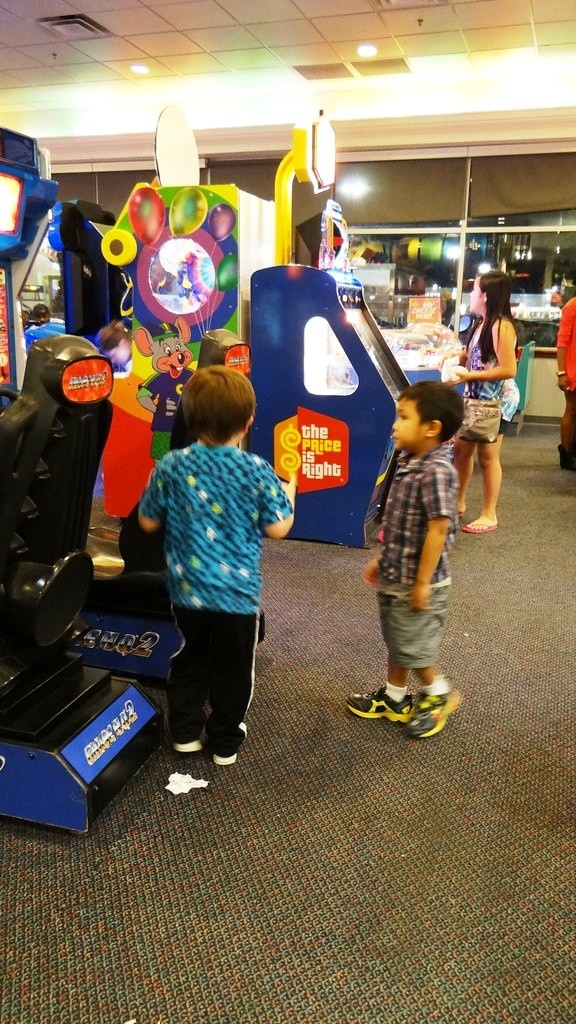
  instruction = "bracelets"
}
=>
[556,371,566,377]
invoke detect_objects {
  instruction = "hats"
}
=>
[33,303,52,317]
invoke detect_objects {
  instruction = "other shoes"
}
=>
[558,444,576,470]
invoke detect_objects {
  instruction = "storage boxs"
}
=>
[408,296,444,326]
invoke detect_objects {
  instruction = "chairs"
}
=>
[511,340,536,436]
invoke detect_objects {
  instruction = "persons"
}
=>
[555,280,576,472]
[21,303,62,325]
[437,270,520,534]
[139,364,296,766]
[346,380,466,740]
[99,321,133,373]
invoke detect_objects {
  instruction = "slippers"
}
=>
[463,521,497,532]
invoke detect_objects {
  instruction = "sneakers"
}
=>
[172,727,206,753]
[346,687,414,723]
[213,722,247,766]
[403,689,460,738]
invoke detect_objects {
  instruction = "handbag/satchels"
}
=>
[459,397,501,443]
